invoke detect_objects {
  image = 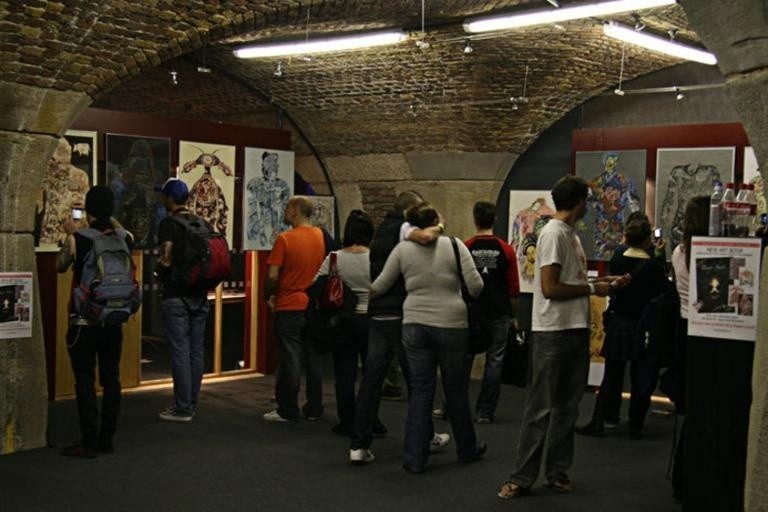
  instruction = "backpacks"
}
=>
[74,227,141,327]
[167,214,231,293]
[634,288,687,369]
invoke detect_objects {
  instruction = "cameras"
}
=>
[71,204,82,221]
[135,230,160,256]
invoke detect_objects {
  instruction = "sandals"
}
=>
[498,482,523,500]
[552,478,574,496]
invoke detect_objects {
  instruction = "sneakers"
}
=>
[380,380,404,402]
[62,439,115,458]
[624,418,645,438]
[431,432,450,450]
[458,439,487,462]
[348,447,377,462]
[263,394,323,423]
[330,417,388,437]
[432,408,450,419]
[158,403,202,422]
[574,416,620,437]
[401,461,427,474]
[476,414,492,424]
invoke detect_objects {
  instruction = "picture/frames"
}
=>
[103,131,171,250]
[32,128,98,253]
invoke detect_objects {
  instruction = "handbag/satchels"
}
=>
[303,252,361,354]
[501,328,529,388]
[468,313,493,354]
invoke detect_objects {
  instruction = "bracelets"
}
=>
[437,224,444,234]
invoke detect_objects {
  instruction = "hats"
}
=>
[84,184,116,221]
[154,177,188,202]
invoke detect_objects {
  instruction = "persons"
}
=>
[348,190,451,463]
[156,177,209,423]
[54,183,134,459]
[262,195,327,422]
[497,174,624,501]
[313,209,390,442]
[369,201,486,473]
[433,201,520,423]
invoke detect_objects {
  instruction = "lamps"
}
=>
[602,18,717,65]
[461,0,675,33]
[232,26,411,64]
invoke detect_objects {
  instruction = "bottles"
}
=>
[708,178,757,237]
